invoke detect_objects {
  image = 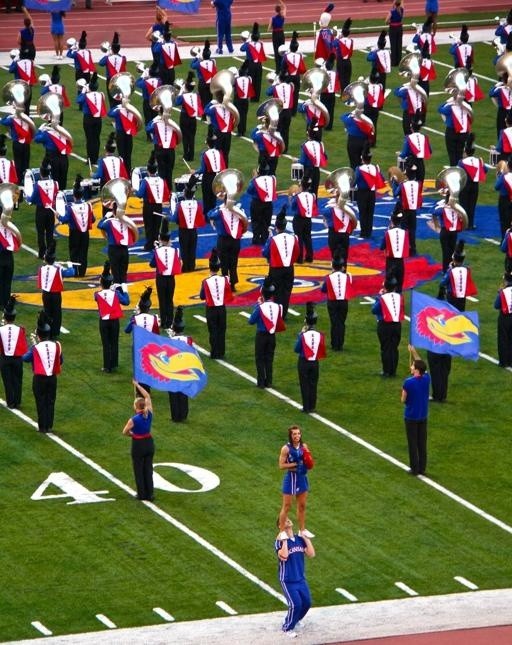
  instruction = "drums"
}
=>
[55,189,82,224]
[23,167,51,206]
[131,167,149,191]
[169,191,196,216]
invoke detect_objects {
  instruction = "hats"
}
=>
[302,170,312,183]
[455,242,465,257]
[423,16,433,29]
[406,158,416,174]
[184,176,196,192]
[259,157,270,170]
[150,57,160,73]
[90,72,99,88]
[252,23,260,37]
[262,276,275,291]
[327,54,336,67]
[101,262,113,280]
[173,306,186,327]
[332,248,344,265]
[52,66,60,80]
[37,310,51,331]
[465,133,475,149]
[79,30,87,45]
[111,31,120,46]
[106,132,117,147]
[40,158,51,170]
[185,72,194,86]
[362,141,371,155]
[460,24,469,39]
[385,270,397,286]
[207,124,217,140]
[240,59,249,72]
[45,239,57,257]
[140,287,152,306]
[392,202,405,220]
[147,150,157,168]
[74,175,84,193]
[160,216,171,236]
[209,248,221,266]
[203,40,211,55]
[309,115,319,134]
[378,31,387,43]
[342,18,352,32]
[305,303,318,320]
[290,32,299,47]
[4,297,17,315]
[413,108,422,122]
[277,209,287,224]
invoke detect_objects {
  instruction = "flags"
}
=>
[157,0,200,14]
[132,323,208,399]
[409,290,481,362]
[24,0,72,12]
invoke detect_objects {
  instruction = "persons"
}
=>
[371,265,404,377]
[340,105,375,170]
[247,153,277,244]
[191,124,226,215]
[274,515,315,638]
[108,100,138,185]
[135,62,163,142]
[75,71,107,166]
[0,134,19,187]
[0,296,28,408]
[232,58,257,137]
[240,22,267,103]
[495,8,512,54]
[279,30,306,116]
[66,30,96,112]
[50,10,66,60]
[268,0,287,75]
[428,189,463,276]
[40,66,71,127]
[319,53,339,130]
[366,29,391,93]
[145,106,178,192]
[250,114,285,177]
[417,40,436,98]
[314,12,335,70]
[493,33,512,66]
[288,168,319,264]
[385,0,404,67]
[400,108,433,182]
[191,40,216,122]
[401,344,430,475]
[0,206,19,310]
[153,20,182,86]
[496,154,512,245]
[33,118,70,191]
[437,96,473,167]
[262,203,300,322]
[200,247,234,359]
[298,88,328,160]
[204,91,234,163]
[17,6,36,61]
[439,240,470,314]
[248,277,286,389]
[211,0,234,54]
[279,426,315,540]
[413,16,437,57]
[494,271,512,367]
[37,239,75,341]
[122,379,156,501]
[97,201,134,286]
[149,219,182,329]
[206,193,245,292]
[130,150,169,251]
[346,66,385,147]
[489,72,512,139]
[25,156,59,259]
[321,244,352,352]
[496,112,512,167]
[332,17,353,98]
[125,287,160,399]
[457,132,489,229]
[318,190,357,275]
[99,32,126,129]
[266,60,295,154]
[0,100,33,188]
[392,160,423,255]
[9,47,37,117]
[163,175,206,272]
[501,220,512,274]
[426,0,438,36]
[427,350,451,401]
[449,24,473,76]
[144,7,168,76]
[465,55,485,156]
[21,311,64,433]
[294,300,325,413]
[174,70,204,162]
[393,72,426,136]
[90,132,128,219]
[293,116,328,194]
[168,306,189,421]
[55,174,95,278]
[94,259,130,373]
[351,140,388,238]
[380,201,409,293]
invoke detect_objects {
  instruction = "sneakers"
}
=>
[279,530,289,540]
[297,529,315,538]
[295,620,304,628]
[284,630,297,638]
